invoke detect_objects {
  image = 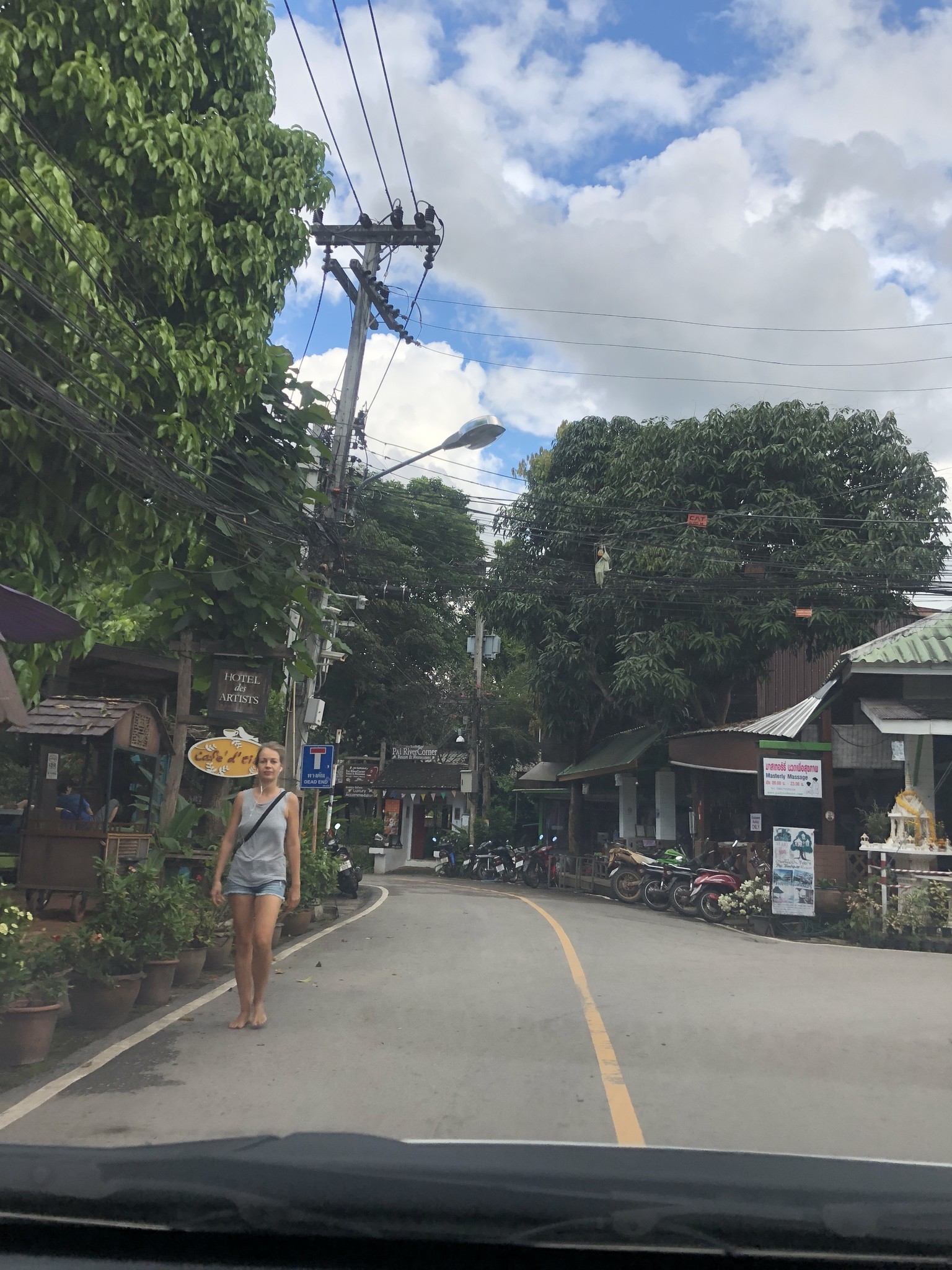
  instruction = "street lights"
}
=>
[284,414,507,798]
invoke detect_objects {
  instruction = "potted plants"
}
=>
[841,877,952,954]
[67,829,343,1028]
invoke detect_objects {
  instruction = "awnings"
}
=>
[857,694,952,735]
[0,584,86,643]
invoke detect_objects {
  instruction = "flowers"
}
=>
[0,880,108,1009]
[716,875,771,916]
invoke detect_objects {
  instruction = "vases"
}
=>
[0,1001,64,1067]
[752,916,776,936]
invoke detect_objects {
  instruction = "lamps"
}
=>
[455,728,465,743]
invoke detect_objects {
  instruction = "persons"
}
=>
[0,800,35,810]
[211,741,301,1028]
[52,778,120,831]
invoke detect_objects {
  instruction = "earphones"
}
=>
[280,767,282,772]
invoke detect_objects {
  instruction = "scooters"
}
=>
[322,823,363,900]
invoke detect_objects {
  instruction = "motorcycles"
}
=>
[432,835,559,889]
[608,837,773,924]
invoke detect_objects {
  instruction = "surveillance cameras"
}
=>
[328,607,342,616]
[359,597,370,606]
[320,650,348,661]
